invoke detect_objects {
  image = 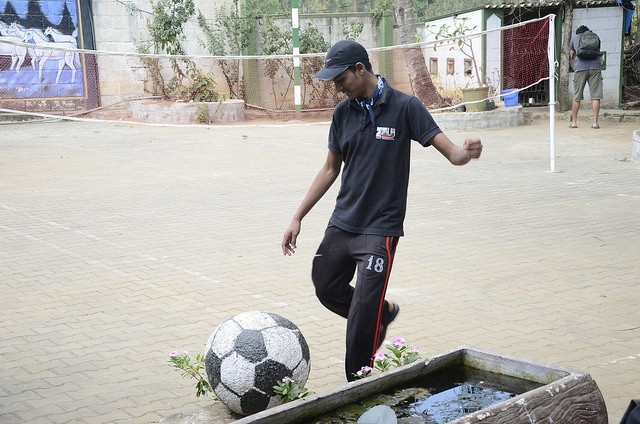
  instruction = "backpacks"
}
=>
[577,30,602,61]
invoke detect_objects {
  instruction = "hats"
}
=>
[312,40,370,81]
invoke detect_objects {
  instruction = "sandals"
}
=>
[377,300,400,350]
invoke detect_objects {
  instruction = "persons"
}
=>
[281,39,482,382]
[569,25,603,129]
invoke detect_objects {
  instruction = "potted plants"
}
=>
[422,14,489,111]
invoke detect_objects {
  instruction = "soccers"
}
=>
[205,310,311,416]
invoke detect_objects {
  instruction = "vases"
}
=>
[487,102,495,109]
[457,105,464,112]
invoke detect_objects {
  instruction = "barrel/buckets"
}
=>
[502,88,519,107]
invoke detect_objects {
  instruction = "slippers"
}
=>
[591,124,600,129]
[569,123,577,128]
